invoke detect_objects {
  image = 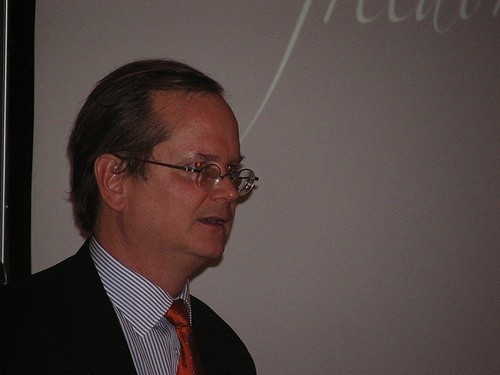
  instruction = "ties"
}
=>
[164,299,199,374]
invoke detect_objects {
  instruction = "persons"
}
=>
[1,59,258,374]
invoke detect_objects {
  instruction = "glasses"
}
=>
[117,156,259,196]
